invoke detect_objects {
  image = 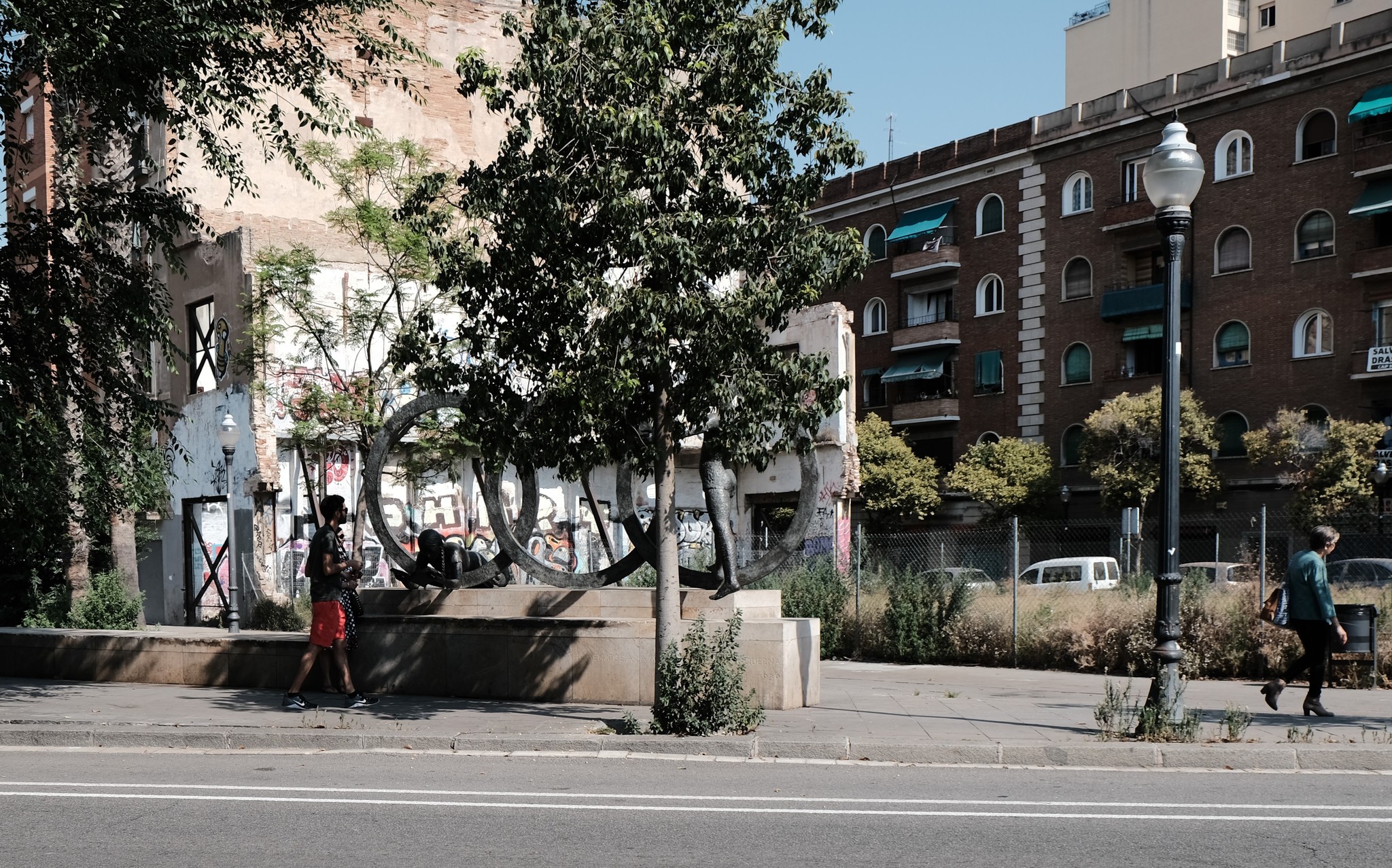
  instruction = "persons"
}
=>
[670,403,741,601]
[281,495,380,710]
[1260,526,1348,718]
[392,528,510,590]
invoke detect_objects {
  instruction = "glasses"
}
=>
[342,507,348,514]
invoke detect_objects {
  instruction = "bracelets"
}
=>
[344,561,348,568]
[345,581,349,588]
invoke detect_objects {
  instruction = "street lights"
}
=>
[218,409,241,633]
[1133,121,1207,738]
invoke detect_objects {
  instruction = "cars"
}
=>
[912,567,998,598]
[1324,558,1392,587]
[1179,560,1251,590]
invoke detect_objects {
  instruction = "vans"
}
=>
[1017,557,1120,594]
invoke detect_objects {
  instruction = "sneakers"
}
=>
[282,692,318,711]
[344,693,379,708]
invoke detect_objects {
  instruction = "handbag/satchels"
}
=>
[1260,581,1295,631]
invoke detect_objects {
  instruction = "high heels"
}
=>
[1260,678,1286,711]
[1303,692,1335,717]
